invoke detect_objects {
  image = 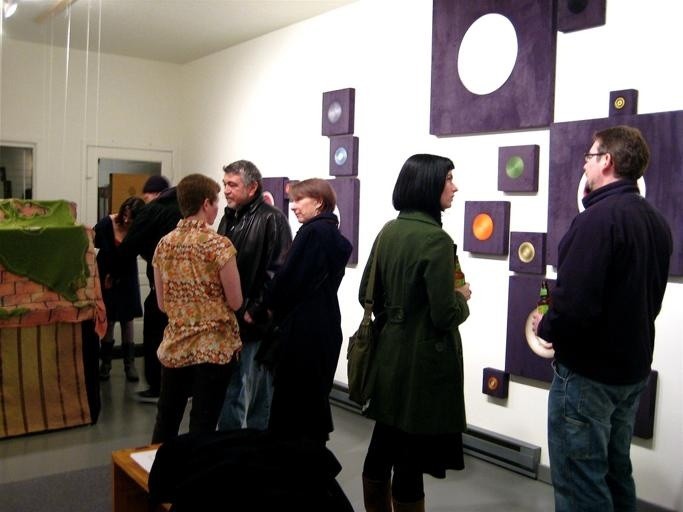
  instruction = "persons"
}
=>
[216,160,293,430]
[266,177,353,445]
[358,155,472,512]
[92,196,145,381]
[151,172,244,446]
[105,175,183,404]
[532,125,673,512]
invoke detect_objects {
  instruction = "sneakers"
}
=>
[131,390,160,404]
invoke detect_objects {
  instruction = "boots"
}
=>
[98,339,115,384]
[122,339,140,381]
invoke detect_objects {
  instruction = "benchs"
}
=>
[110,443,171,511]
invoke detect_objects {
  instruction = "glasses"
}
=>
[583,150,603,159]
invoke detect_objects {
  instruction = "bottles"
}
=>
[455,256,465,288]
[538,280,551,315]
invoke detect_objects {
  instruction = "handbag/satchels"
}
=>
[346,232,381,406]
